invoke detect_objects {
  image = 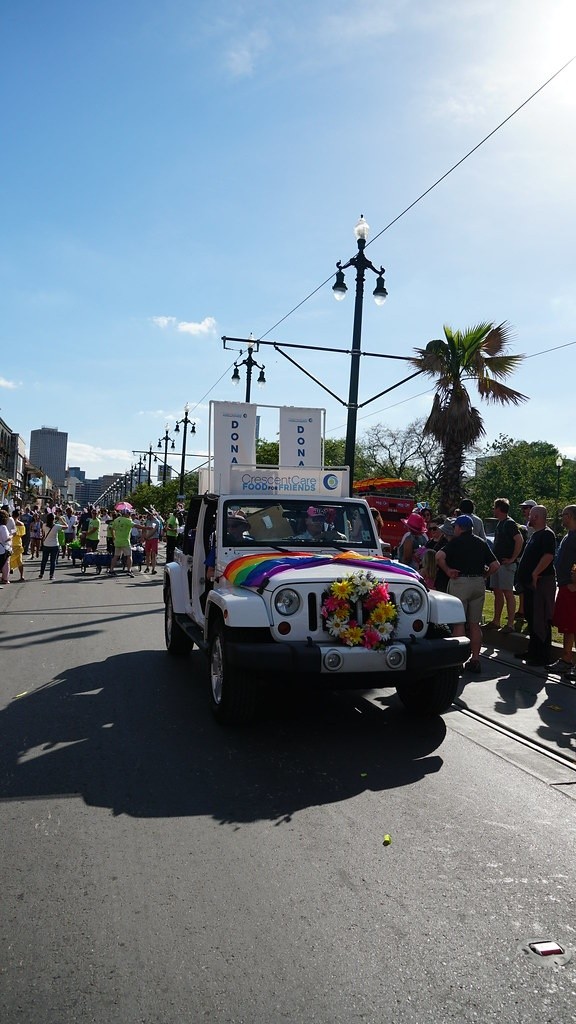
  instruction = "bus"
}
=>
[352,477,417,547]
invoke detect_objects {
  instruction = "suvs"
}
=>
[161,493,474,727]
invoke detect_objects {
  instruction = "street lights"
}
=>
[231,333,268,404]
[329,211,389,497]
[555,456,563,508]
[94,400,196,525]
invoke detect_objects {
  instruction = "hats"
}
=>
[226,514,249,532]
[400,513,428,533]
[306,507,325,516]
[121,509,132,515]
[518,499,537,508]
[438,516,473,537]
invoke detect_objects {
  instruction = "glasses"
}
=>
[429,527,438,531]
[520,507,531,510]
[452,524,458,529]
[308,517,325,522]
[559,513,567,517]
[422,514,430,518]
[226,523,241,529]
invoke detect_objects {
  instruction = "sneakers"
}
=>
[544,659,572,672]
[564,664,576,679]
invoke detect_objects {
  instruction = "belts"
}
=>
[457,573,481,577]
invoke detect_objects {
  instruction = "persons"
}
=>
[397,498,576,672]
[296,506,347,540]
[199,515,254,616]
[347,507,384,542]
[0,501,188,584]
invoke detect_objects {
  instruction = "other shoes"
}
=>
[4,551,157,590]
[528,659,552,666]
[515,649,530,659]
[505,611,525,621]
[464,658,480,673]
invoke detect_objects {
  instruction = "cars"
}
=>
[376,537,393,559]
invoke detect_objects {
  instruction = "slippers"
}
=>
[500,625,516,633]
[482,621,500,630]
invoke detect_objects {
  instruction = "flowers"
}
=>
[320,571,397,651]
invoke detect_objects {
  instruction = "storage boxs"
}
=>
[246,503,294,540]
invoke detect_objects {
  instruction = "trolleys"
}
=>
[72,547,145,574]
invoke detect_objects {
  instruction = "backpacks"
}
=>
[504,518,527,558]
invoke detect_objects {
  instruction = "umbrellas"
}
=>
[114,502,133,514]
